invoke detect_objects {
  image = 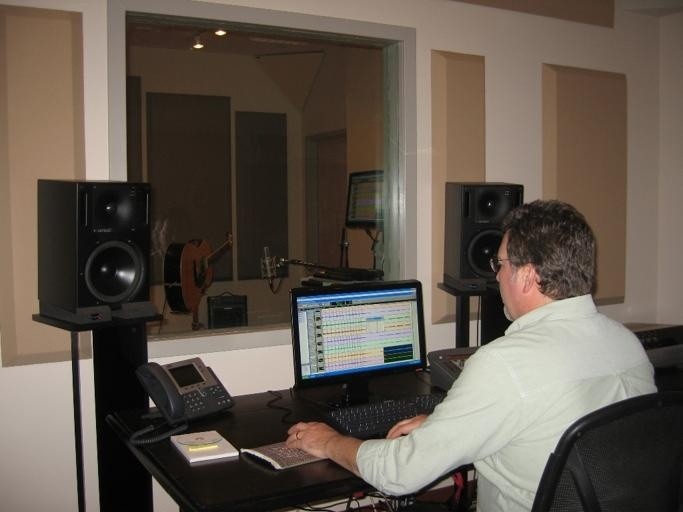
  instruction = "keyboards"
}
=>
[324,392,454,438]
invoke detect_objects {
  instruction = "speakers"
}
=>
[443,181,525,293]
[31,178,165,332]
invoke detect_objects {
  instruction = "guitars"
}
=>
[163,233,233,315]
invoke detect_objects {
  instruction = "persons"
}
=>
[287,199,658,512]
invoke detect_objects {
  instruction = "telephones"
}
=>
[129,357,235,445]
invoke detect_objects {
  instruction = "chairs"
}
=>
[529,390,682,511]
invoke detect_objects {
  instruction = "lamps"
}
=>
[216,28,226,38]
[190,33,204,49]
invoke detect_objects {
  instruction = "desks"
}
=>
[107,362,484,511]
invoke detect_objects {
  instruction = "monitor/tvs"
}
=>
[289,279,427,409]
[346,170,388,228]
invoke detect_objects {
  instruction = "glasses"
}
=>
[490,256,514,273]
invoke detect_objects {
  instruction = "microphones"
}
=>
[260,246,279,283]
